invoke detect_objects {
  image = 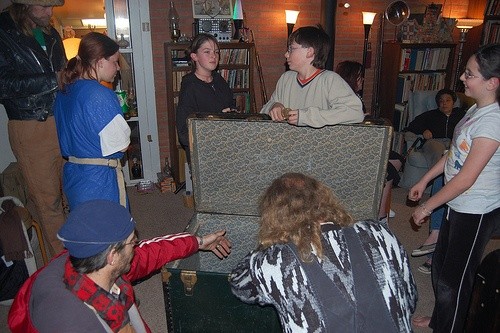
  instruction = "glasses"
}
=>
[462,65,481,81]
[287,46,304,53]
[122,233,138,247]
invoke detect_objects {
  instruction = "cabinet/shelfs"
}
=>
[164,42,255,183]
[378,43,457,119]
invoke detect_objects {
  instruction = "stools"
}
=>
[15,206,48,265]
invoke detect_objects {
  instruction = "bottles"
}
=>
[131,157,142,179]
[168,0,179,30]
[127,91,137,116]
[163,157,172,178]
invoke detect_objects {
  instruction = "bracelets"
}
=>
[200,235,203,245]
[420,202,433,215]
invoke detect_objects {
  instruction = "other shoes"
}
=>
[417,259,431,274]
[390,208,396,218]
[380,214,390,228]
[412,242,437,256]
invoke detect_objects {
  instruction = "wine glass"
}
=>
[170,29,181,44]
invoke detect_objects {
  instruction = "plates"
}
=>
[173,61,188,63]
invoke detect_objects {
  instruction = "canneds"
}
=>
[115,89,128,113]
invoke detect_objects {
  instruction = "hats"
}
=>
[12,0,67,6]
[56,201,134,258]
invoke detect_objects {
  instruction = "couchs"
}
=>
[398,90,461,195]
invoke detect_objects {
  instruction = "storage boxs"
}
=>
[161,112,394,333]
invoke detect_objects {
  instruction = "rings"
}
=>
[216,244,220,248]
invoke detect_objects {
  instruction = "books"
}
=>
[391,47,451,155]
[480,0,500,47]
[217,48,251,114]
[172,51,192,108]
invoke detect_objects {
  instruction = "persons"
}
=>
[336,60,404,225]
[175,33,241,169]
[227,172,418,333]
[407,89,467,274]
[7,198,232,333]
[260,25,365,129]
[0,0,68,258]
[53,31,131,214]
[409,44,500,333]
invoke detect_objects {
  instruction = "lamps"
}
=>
[453,26,473,93]
[360,12,377,97]
[285,10,300,71]
[169,1,181,42]
